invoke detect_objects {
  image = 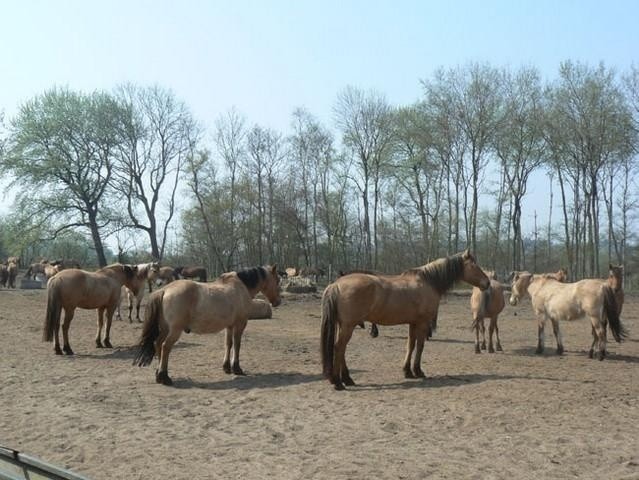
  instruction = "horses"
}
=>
[319,248,491,391]
[278,263,324,289]
[0,255,208,289]
[132,262,282,386]
[114,260,163,324]
[469,268,506,353]
[42,262,140,355]
[508,262,629,362]
